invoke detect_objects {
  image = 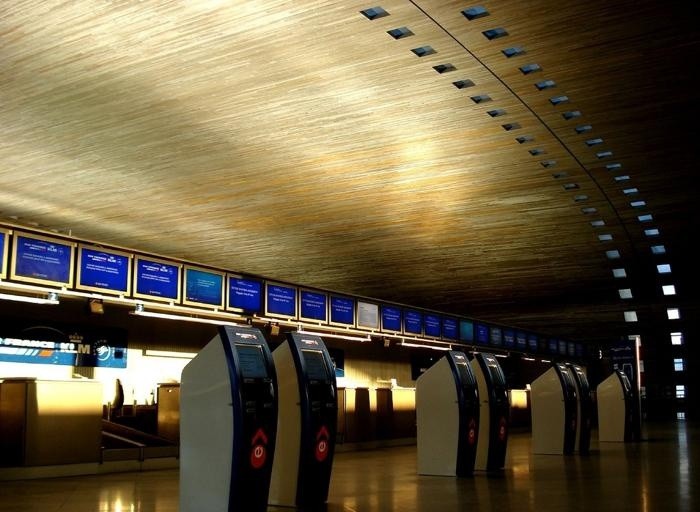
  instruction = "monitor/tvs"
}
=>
[133,254,182,304]
[226,273,264,316]
[265,280,298,320]
[381,304,583,355]
[622,376,631,391]
[561,370,588,392]
[75,242,131,297]
[235,342,268,379]
[0,227,9,279]
[356,299,381,332]
[329,293,355,328]
[489,366,502,384]
[299,286,328,325]
[183,263,225,310]
[456,362,473,384]
[301,349,330,381]
[10,229,75,288]
